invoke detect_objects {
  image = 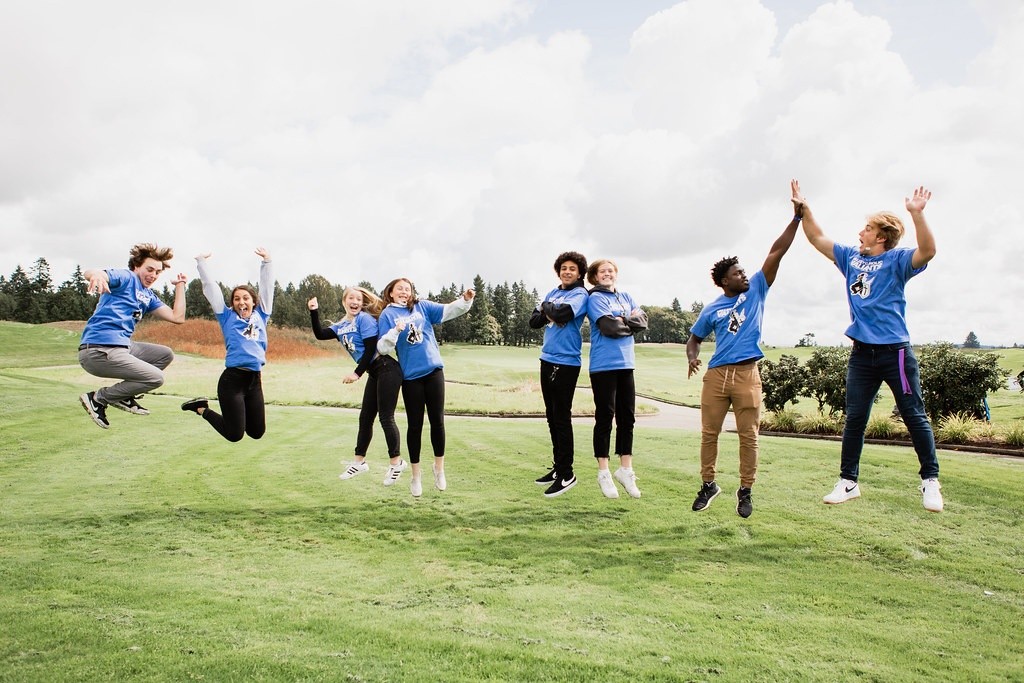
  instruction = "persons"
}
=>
[182,245,276,443]
[684,177,807,519]
[78,243,188,430]
[791,178,945,514]
[587,258,649,500]
[307,285,408,486]
[529,252,589,497]
[376,278,476,498]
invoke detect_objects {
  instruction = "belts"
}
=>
[78,344,128,351]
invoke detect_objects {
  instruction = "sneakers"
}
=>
[692,481,721,511]
[598,469,619,499]
[181,397,208,415]
[383,460,407,485]
[822,476,861,505]
[535,462,558,485]
[109,395,150,415]
[339,461,369,480]
[433,463,446,491]
[411,472,422,496]
[736,486,752,518]
[614,468,641,499]
[544,474,577,497]
[917,478,943,512]
[79,391,110,429]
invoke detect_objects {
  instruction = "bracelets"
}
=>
[175,280,186,284]
[794,217,801,221]
